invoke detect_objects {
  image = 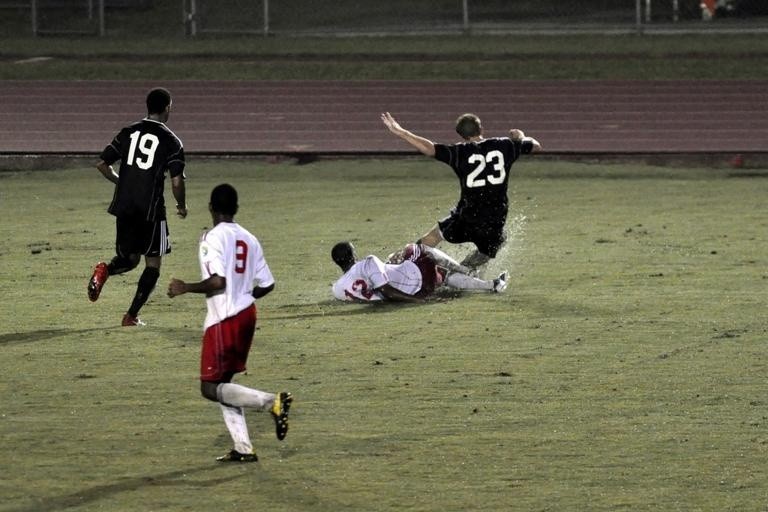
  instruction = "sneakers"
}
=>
[122,313,146,326]
[216,448,258,461]
[494,270,510,292]
[87,262,110,301]
[468,268,480,276]
[270,392,293,440]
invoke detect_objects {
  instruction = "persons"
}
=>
[327,239,513,305]
[86,86,189,327]
[166,182,294,464]
[380,108,546,278]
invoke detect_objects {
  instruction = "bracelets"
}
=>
[175,203,185,209]
